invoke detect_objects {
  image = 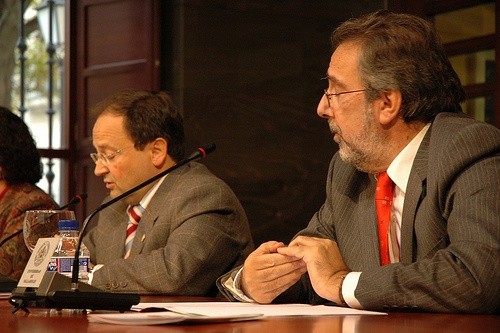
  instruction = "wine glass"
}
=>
[24,210,75,253]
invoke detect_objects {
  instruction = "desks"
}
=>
[0,296,500,333]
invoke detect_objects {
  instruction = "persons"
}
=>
[0,105,66,289]
[79,90,254,297]
[216,13,500,313]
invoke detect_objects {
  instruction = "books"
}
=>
[87,310,264,326]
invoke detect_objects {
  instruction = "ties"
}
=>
[123,205,143,259]
[374,172,396,266]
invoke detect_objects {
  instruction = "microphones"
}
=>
[70,142,217,292]
[0,191,87,248]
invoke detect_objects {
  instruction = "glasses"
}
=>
[324,87,375,106]
[89,142,138,166]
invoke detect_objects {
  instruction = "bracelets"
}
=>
[339,270,353,308]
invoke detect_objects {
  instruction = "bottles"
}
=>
[49,220,90,284]
